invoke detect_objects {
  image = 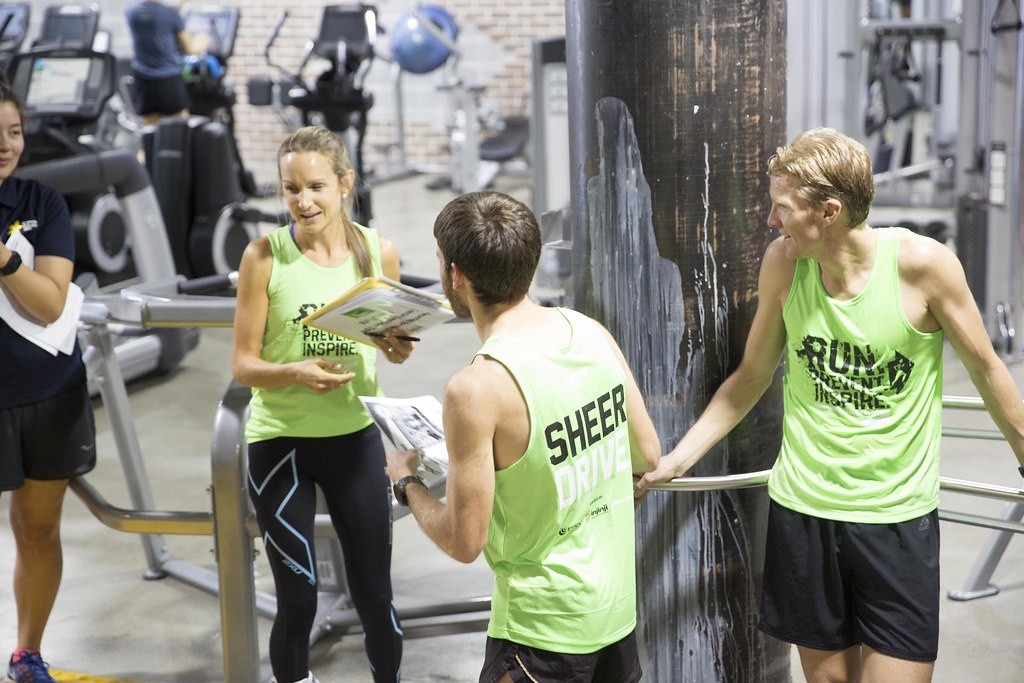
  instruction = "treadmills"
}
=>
[1,39,202,422]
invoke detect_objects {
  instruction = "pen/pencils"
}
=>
[361,330,420,343]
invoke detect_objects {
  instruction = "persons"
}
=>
[386,191,662,682]
[1,83,96,683]
[126,0,210,122]
[630,127,1024,683]
[233,125,415,682]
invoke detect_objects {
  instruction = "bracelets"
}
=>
[1018,465,1024,479]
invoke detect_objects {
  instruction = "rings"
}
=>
[387,347,393,353]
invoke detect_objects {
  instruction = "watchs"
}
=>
[1,250,22,276]
[394,476,429,507]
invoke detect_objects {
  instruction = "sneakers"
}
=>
[7,655,55,683]
